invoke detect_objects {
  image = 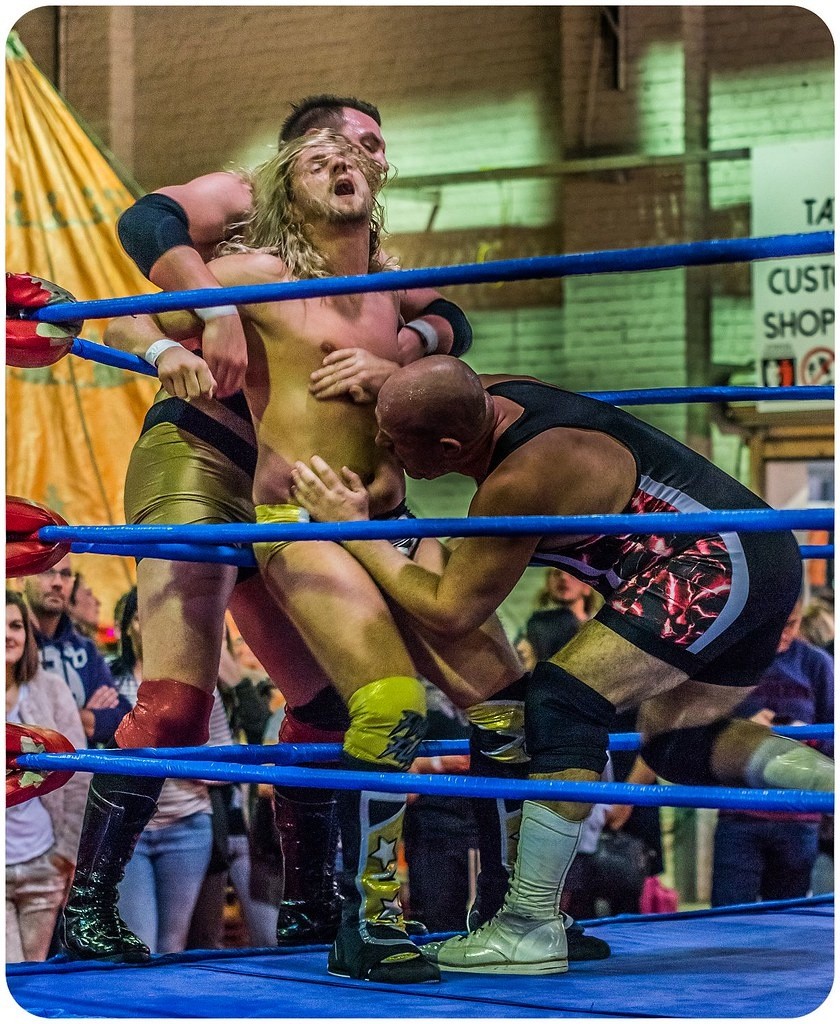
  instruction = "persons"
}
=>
[89,586,239,955]
[189,562,835,952]
[47,93,474,972]
[291,347,835,976]
[7,589,91,965]
[23,554,133,748]
[102,128,610,985]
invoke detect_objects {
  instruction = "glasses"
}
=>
[32,566,80,585]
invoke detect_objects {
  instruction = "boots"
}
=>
[267,760,432,945]
[458,680,609,965]
[37,731,156,961]
[412,795,581,981]
[731,728,833,851]
[324,748,442,984]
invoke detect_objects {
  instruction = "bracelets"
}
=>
[144,339,184,367]
[404,319,440,356]
